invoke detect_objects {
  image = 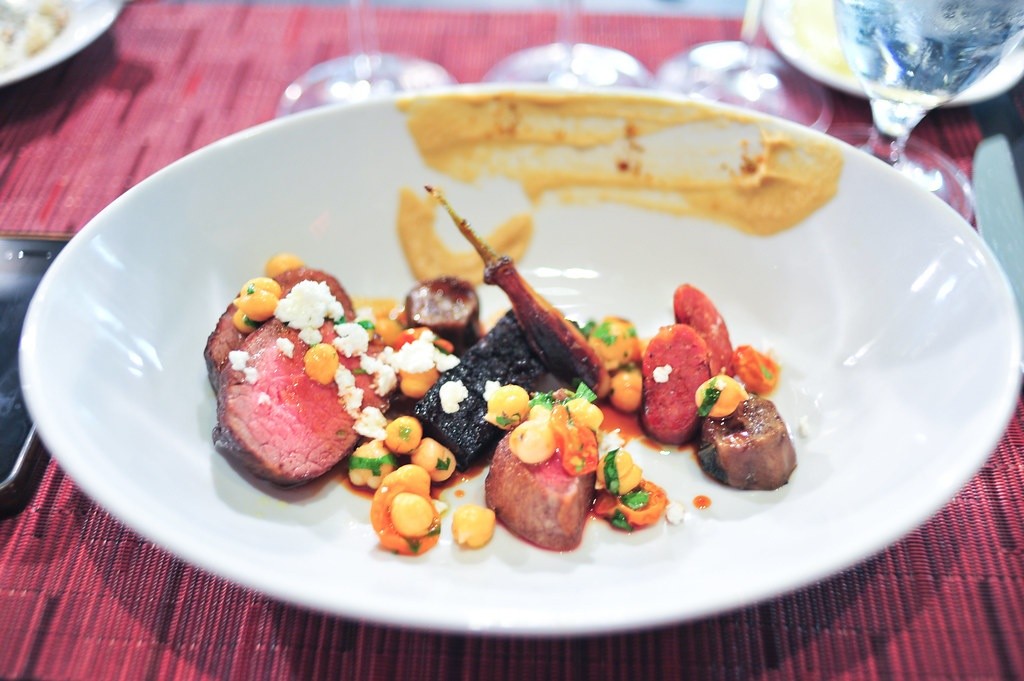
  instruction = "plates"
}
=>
[0,0,126,89]
[764,0,1024,107]
[18,86,1022,635]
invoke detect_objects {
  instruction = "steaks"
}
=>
[199,265,398,492]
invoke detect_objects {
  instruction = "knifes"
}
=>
[972,132,1023,385]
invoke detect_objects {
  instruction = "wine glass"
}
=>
[761,0,1024,225]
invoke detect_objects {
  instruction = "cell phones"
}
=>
[0,229,76,506]
